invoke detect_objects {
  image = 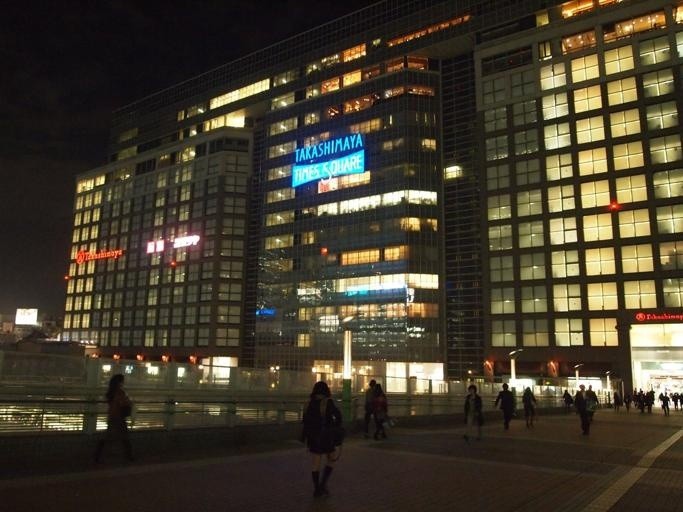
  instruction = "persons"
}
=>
[461,384,482,440]
[614,388,682,416]
[575,384,590,435]
[369,383,388,438]
[494,383,514,430]
[562,389,571,415]
[301,381,343,499]
[586,384,598,421]
[360,378,376,439]
[522,387,537,428]
[92,373,133,466]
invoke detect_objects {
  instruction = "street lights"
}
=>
[342,312,360,431]
[509,349,524,415]
[574,363,611,406]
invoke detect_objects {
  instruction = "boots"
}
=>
[311,466,332,496]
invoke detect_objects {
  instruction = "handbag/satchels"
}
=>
[530,398,536,409]
[382,417,401,435]
[586,400,598,412]
[330,422,344,446]
